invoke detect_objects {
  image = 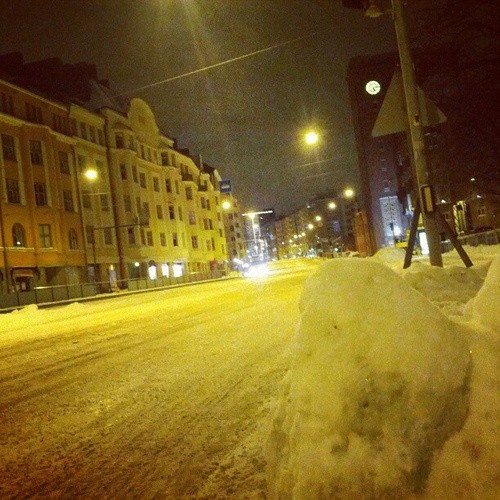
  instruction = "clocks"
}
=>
[365,80,381,96]
